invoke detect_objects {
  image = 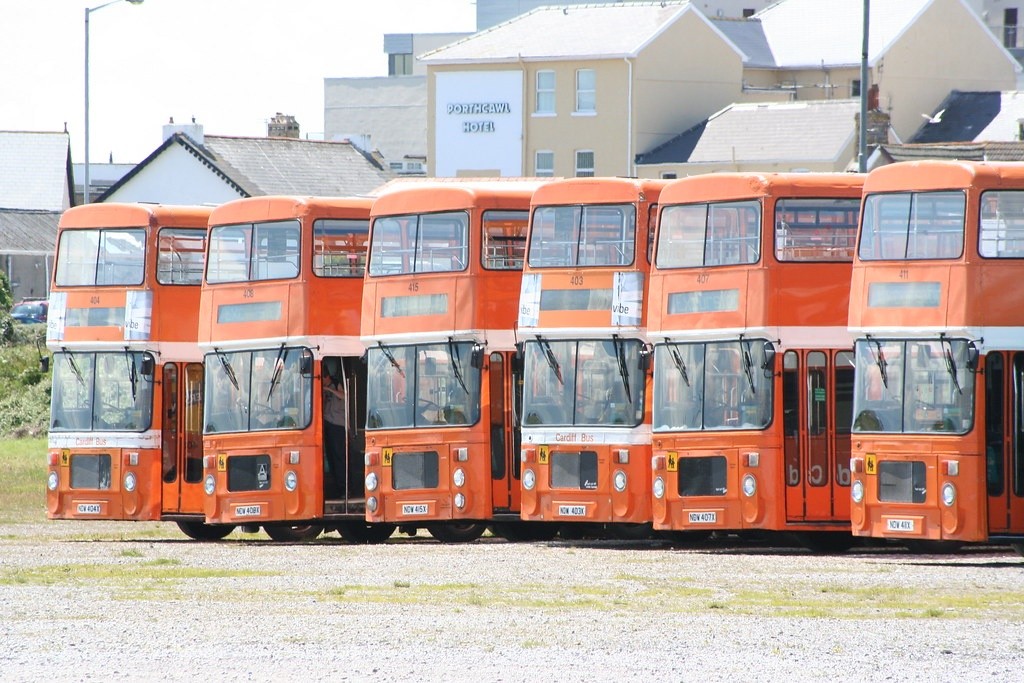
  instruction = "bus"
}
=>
[46,157,1024,544]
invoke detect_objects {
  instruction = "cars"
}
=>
[10,315,42,325]
[9,299,48,317]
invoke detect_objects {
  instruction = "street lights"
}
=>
[84,0,144,204]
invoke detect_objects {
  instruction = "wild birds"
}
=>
[920,108,946,124]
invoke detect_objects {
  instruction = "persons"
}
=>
[321,365,349,499]
